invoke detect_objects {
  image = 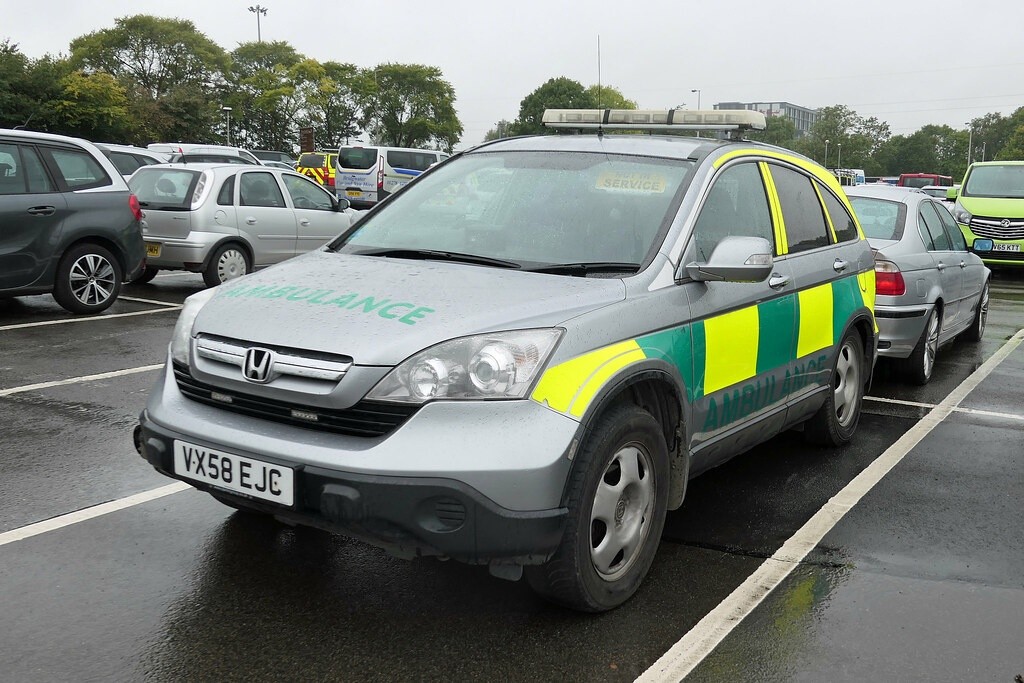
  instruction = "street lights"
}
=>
[837,143,842,184]
[982,141,986,162]
[223,106,232,146]
[692,88,701,110]
[965,122,972,167]
[825,139,830,169]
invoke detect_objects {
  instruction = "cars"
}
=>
[133,106,881,615]
[920,184,960,223]
[0,129,149,318]
[832,183,993,386]
[125,163,359,289]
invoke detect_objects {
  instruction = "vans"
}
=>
[334,144,453,210]
[946,160,1024,272]
[296,151,338,192]
[51,142,298,187]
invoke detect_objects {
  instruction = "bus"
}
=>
[898,173,954,188]
